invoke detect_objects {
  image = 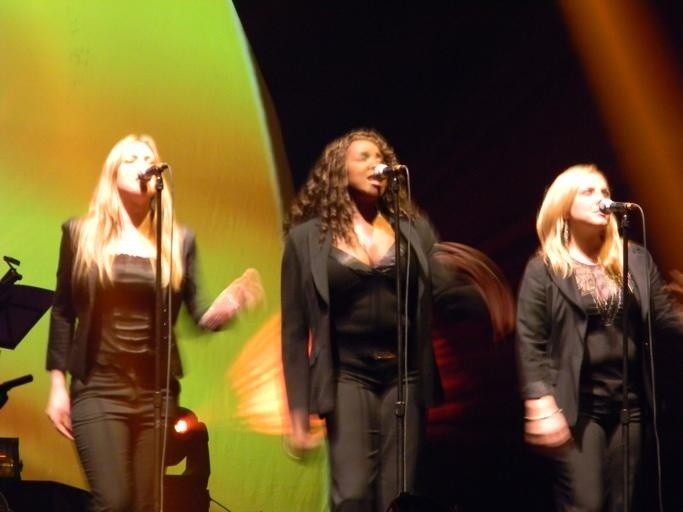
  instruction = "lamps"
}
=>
[161,406,209,510]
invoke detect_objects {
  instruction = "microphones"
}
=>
[136,162,171,180]
[374,163,409,182]
[599,198,642,216]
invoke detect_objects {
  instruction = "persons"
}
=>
[280,127,445,512]
[513,161,682,512]
[44,131,265,509]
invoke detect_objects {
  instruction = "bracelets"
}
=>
[521,408,563,422]
